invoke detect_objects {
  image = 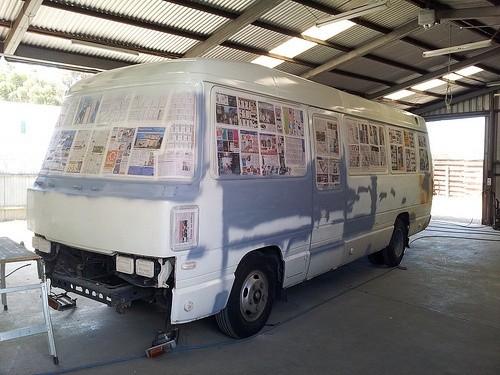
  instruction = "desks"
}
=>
[0,237,60,365]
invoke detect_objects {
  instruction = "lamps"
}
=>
[422,27,500,59]
[300,0,390,29]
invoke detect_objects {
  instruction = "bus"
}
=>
[27,56,435,340]
